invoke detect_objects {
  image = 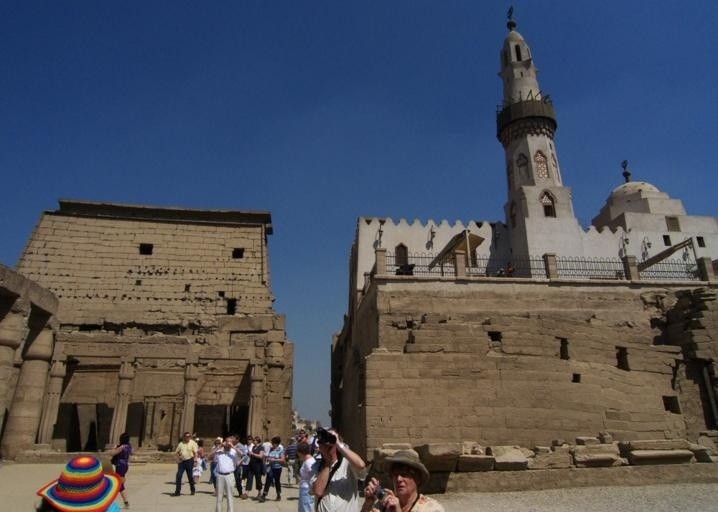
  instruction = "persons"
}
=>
[361,450,445,512]
[110,433,132,508]
[170,427,367,512]
[496,268,505,277]
[506,262,515,277]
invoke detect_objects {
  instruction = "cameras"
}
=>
[374,487,386,501]
[316,426,337,446]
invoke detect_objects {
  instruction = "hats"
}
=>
[383,449,430,492]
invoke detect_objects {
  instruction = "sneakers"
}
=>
[239,494,281,502]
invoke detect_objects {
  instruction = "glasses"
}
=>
[390,469,415,478]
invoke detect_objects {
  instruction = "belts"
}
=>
[218,472,233,475]
